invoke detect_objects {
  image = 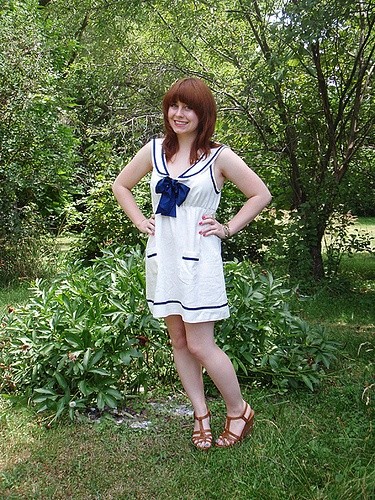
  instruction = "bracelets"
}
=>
[222,224,231,240]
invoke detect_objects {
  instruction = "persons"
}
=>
[110,77,273,449]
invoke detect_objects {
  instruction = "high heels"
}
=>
[215,402,254,448]
[191,404,212,450]
